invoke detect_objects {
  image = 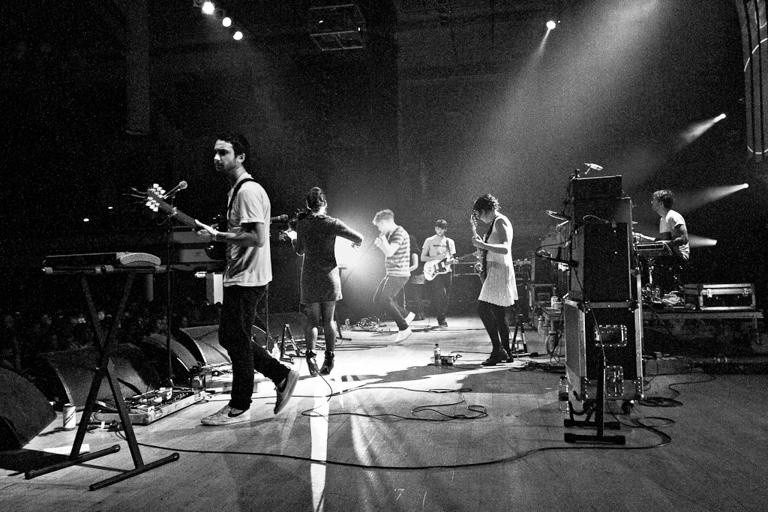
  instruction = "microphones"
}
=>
[161,178,189,201]
[271,215,288,223]
[446,239,450,251]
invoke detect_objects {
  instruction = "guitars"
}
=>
[423,248,483,281]
[122,183,227,261]
[470,214,487,284]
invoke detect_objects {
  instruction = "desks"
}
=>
[25,264,179,490]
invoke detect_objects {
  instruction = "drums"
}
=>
[638,243,664,251]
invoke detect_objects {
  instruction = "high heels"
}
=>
[320,352,335,375]
[305,350,319,376]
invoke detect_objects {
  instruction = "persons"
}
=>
[420,219,456,328]
[191,134,299,427]
[470,193,518,366]
[372,209,415,344]
[287,185,363,377]
[0,294,223,381]
[404,234,426,320]
[633,189,690,294]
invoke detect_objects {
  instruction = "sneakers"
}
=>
[273,367,298,413]
[395,326,412,344]
[506,351,514,363]
[405,312,416,326]
[201,406,250,427]
[483,348,509,366]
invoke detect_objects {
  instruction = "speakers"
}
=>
[568,222,630,301]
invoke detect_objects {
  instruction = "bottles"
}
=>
[434,343,441,366]
[558,377,569,412]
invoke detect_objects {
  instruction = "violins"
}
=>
[278,210,309,242]
